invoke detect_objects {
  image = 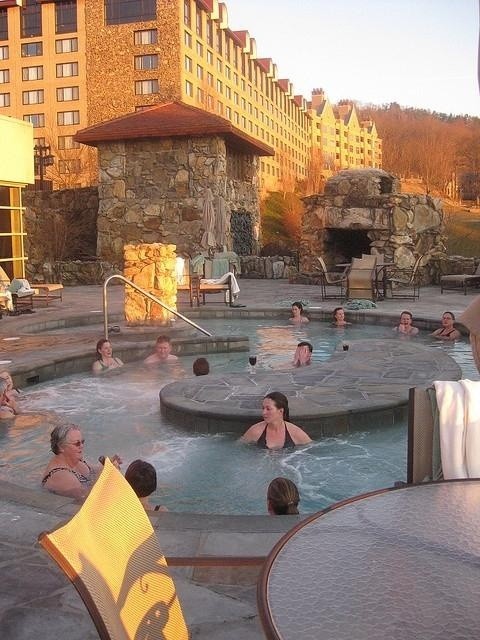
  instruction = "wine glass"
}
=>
[248,355,258,375]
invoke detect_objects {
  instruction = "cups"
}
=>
[341,340,350,352]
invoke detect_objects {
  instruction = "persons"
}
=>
[238,392,312,450]
[40,423,122,502]
[391,311,419,336]
[331,307,353,326]
[142,335,178,363]
[125,459,170,511]
[0,377,21,419]
[432,312,460,338]
[0,369,26,395]
[288,302,309,322]
[265,477,301,515]
[279,342,313,368]
[192,356,210,375]
[91,339,125,374]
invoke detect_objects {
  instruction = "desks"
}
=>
[258,477,480,640]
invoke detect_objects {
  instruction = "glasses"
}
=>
[67,440,85,446]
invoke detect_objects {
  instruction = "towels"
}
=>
[432,379,480,480]
[201,272,240,302]
[176,257,185,285]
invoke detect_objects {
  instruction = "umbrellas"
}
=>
[201,188,218,260]
[215,197,225,252]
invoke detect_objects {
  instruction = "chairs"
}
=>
[176,259,193,307]
[196,260,233,306]
[317,253,425,302]
[439,262,480,295]
[38,455,267,640]
[407,386,435,483]
[0,265,64,316]
